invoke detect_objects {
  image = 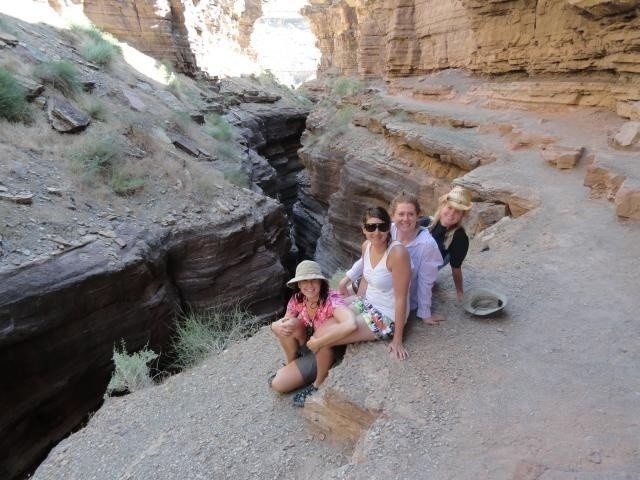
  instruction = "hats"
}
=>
[286,259,329,290]
[465,289,507,318]
[447,185,473,211]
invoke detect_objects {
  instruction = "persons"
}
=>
[389,185,470,326]
[270,206,412,408]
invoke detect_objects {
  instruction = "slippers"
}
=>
[293,385,316,408]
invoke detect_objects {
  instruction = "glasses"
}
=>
[365,223,388,232]
